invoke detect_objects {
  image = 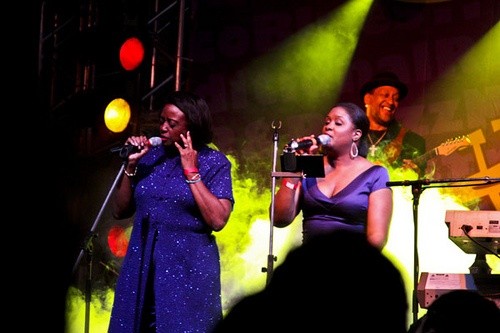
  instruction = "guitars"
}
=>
[395,132,473,171]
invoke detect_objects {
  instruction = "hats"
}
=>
[359,70,407,102]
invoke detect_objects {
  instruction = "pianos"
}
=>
[444,209,500,254]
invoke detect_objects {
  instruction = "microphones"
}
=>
[291,134,332,150]
[123,136,162,154]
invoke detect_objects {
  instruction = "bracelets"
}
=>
[282,179,299,191]
[123,165,138,177]
[183,167,201,183]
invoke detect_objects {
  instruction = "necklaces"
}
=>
[366,131,388,156]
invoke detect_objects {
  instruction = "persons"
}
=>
[105,91,235,333]
[269,102,393,252]
[348,70,427,181]
[265,236,407,332]
[407,289,500,333]
[211,290,267,332]
